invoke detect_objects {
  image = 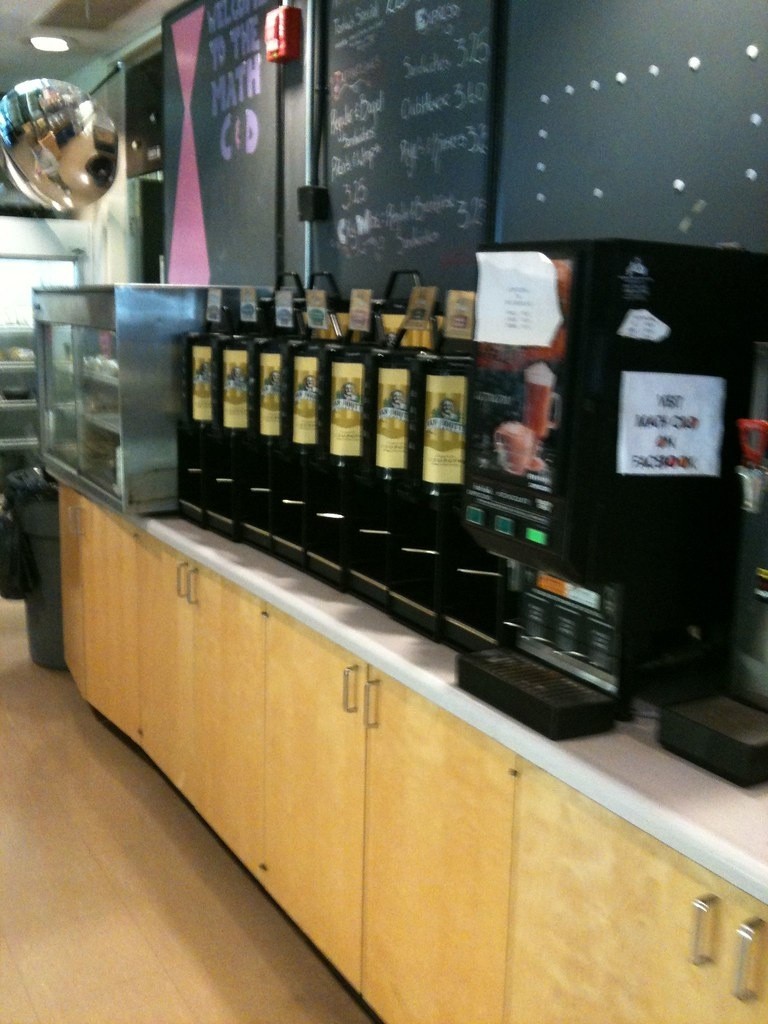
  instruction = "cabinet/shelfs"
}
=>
[58,484,768,1024]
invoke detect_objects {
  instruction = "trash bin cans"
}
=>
[37,130,63,160]
[7,466,59,671]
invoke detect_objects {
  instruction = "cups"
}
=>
[523,367,561,439]
[495,424,538,475]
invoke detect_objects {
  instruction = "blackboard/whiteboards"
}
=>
[318,0,768,310]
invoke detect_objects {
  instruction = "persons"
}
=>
[28,136,71,195]
